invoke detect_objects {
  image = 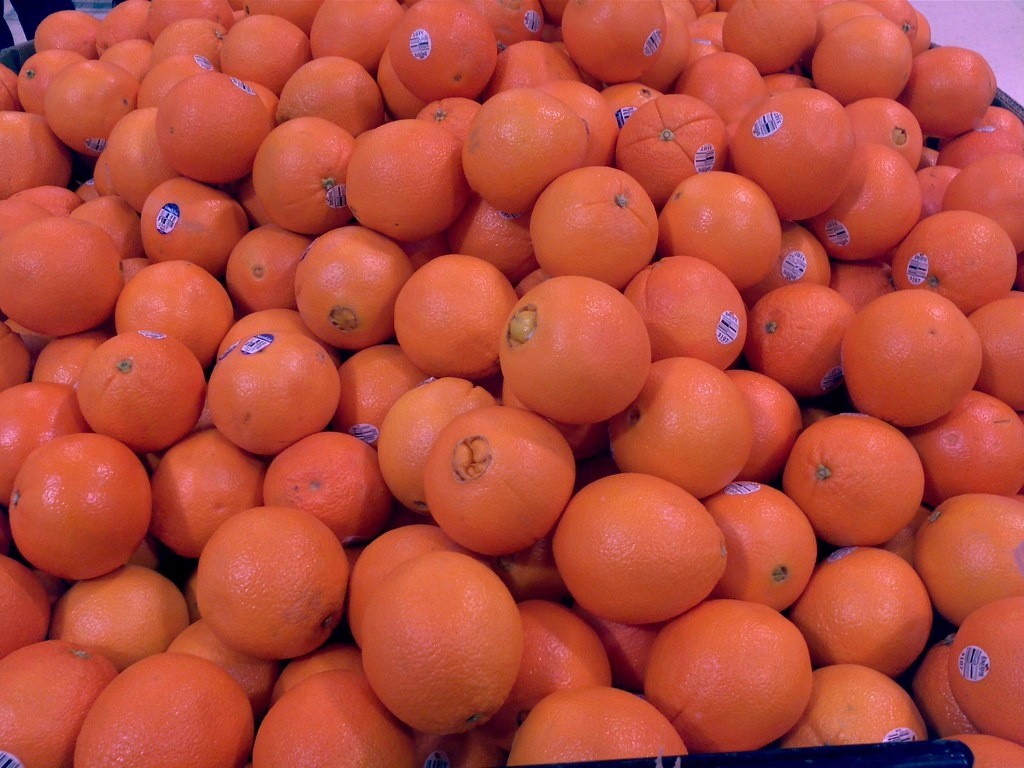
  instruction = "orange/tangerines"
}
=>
[0,0,1024,768]
